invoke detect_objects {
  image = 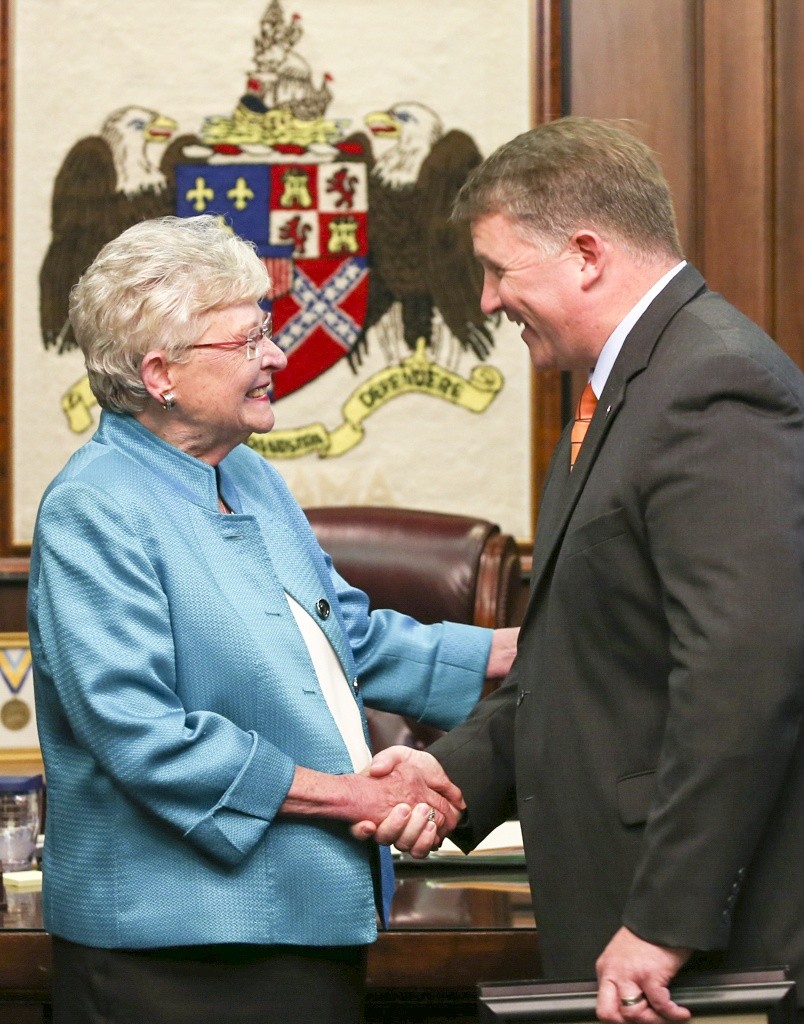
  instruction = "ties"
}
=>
[571,381,599,471]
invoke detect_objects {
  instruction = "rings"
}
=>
[428,808,436,821]
[620,992,645,1006]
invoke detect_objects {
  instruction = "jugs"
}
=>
[0,774,43,905]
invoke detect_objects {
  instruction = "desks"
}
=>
[0,879,543,1024]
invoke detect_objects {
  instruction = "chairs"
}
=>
[294,506,521,759]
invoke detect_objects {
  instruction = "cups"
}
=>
[3,870,42,915]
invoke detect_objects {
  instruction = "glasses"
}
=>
[135,312,272,369]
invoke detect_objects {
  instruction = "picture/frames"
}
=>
[0,0,564,588]
[0,632,43,763]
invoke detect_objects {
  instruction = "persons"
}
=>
[25,214,521,1024]
[350,114,804,1024]
